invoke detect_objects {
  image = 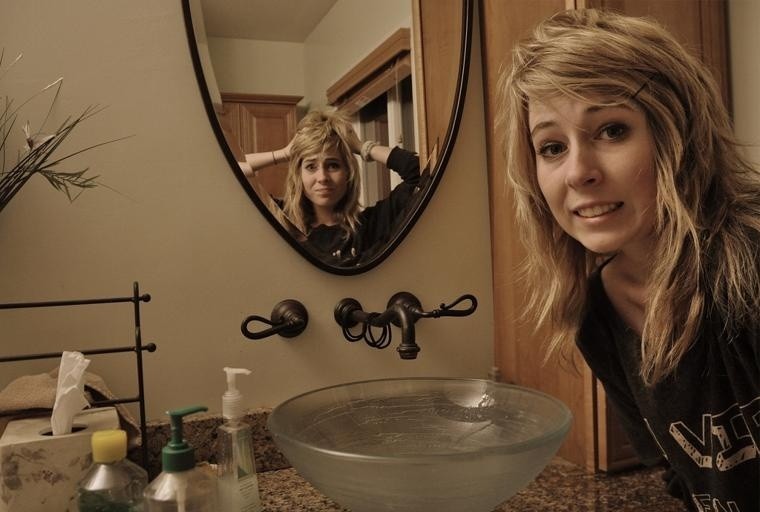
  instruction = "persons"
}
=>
[490,5,759,511]
[234,105,422,269]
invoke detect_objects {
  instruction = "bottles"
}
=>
[76,428,150,512]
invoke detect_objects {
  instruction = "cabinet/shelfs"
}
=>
[218,91,305,202]
[480,0,736,482]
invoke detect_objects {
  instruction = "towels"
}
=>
[0,367,142,455]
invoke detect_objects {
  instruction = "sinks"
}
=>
[267,379,572,511]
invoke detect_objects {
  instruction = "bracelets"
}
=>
[359,140,378,163]
[270,150,278,165]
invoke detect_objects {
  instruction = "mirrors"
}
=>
[180,0,473,277]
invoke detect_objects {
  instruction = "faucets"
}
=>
[334,298,420,360]
